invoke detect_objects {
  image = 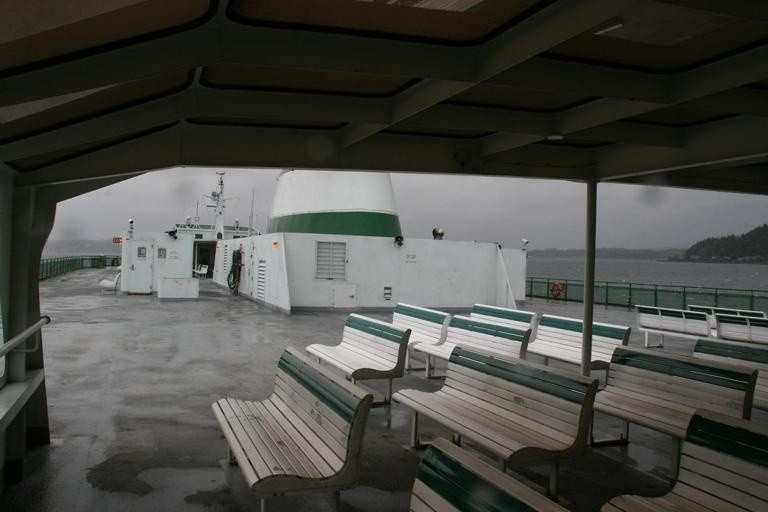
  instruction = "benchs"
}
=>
[392,343,599,501]
[393,303,452,370]
[599,407,768,512]
[212,347,373,512]
[413,314,531,379]
[410,437,568,512]
[306,313,411,428]
